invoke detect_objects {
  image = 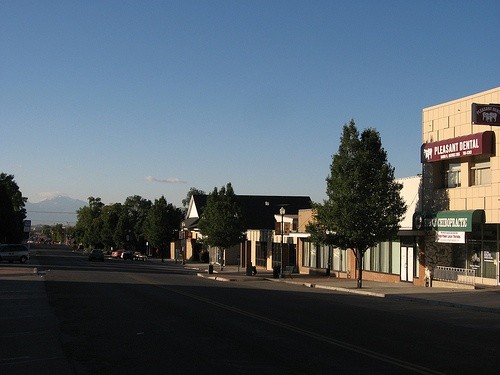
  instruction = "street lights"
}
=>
[280,207,286,277]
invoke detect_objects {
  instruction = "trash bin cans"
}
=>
[208,264,214,274]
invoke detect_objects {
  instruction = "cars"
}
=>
[88,249,105,263]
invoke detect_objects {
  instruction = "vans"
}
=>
[0,244,29,264]
